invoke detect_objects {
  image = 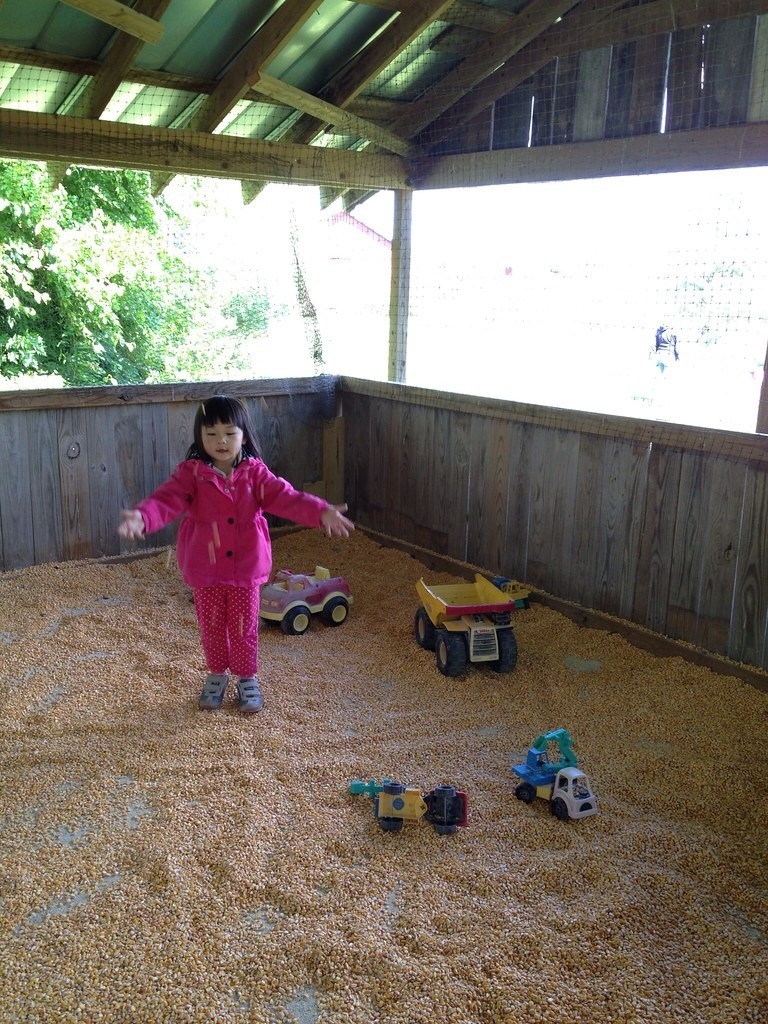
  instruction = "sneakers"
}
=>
[237,678,262,713]
[199,673,229,708]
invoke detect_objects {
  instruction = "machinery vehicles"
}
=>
[512,728,599,820]
[348,779,470,834]
[414,572,521,677]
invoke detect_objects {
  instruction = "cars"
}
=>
[259,566,352,633]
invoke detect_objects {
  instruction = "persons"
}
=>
[654,321,680,373]
[117,395,354,713]
